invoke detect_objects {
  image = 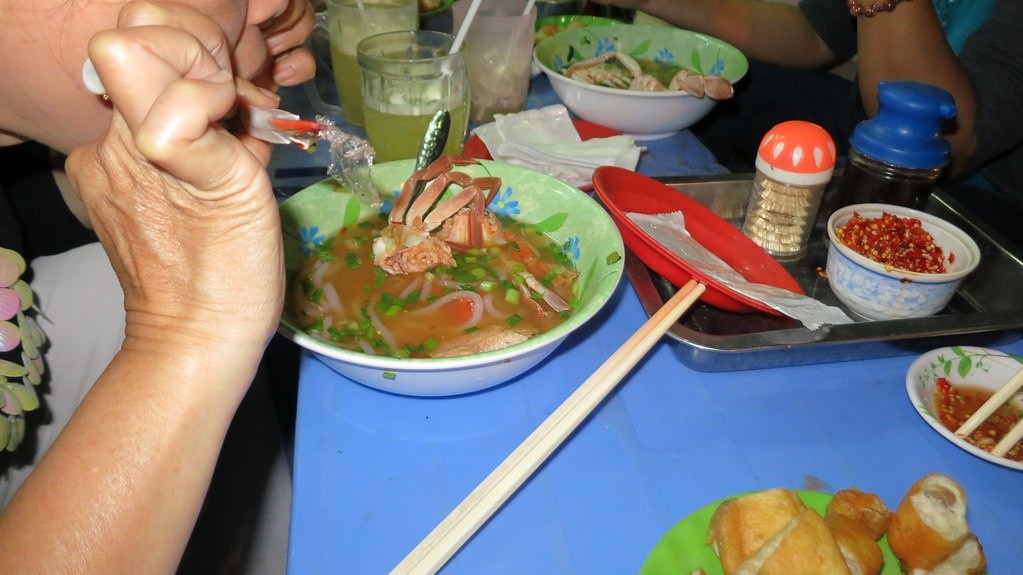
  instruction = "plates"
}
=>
[638,489,905,575]
[906,344,1023,476]
[531,13,628,29]
[590,163,807,317]
[461,118,639,191]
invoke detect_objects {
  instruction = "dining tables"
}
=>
[0,63,1020,573]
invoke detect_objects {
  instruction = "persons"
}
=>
[0,0,318,573]
[605,0,1023,167]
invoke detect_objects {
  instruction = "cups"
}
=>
[450,0,535,126]
[297,1,420,127]
[357,29,471,163]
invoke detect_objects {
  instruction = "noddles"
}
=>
[296,208,578,362]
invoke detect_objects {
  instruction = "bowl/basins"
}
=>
[825,199,983,322]
[266,156,625,399]
[533,28,752,142]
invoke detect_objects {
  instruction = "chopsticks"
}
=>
[381,278,705,575]
[954,364,1023,460]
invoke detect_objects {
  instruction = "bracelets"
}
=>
[848,0,912,18]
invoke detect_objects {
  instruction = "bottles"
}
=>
[824,76,958,225]
[741,121,837,259]
